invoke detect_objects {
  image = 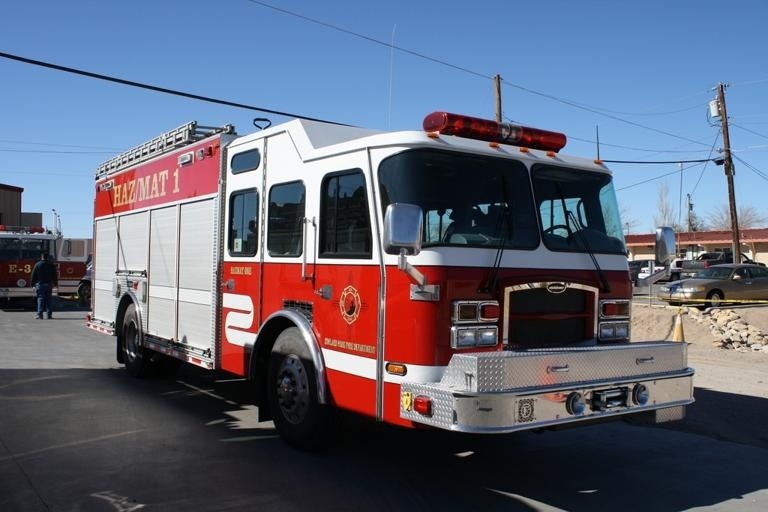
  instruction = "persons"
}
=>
[30,251,59,319]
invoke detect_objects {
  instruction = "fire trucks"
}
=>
[0,224,93,312]
[85,111,697,453]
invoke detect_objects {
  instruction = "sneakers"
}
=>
[34,314,43,320]
[47,316,53,320]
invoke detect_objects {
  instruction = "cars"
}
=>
[627,251,768,307]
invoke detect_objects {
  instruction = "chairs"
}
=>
[246,202,512,253]
